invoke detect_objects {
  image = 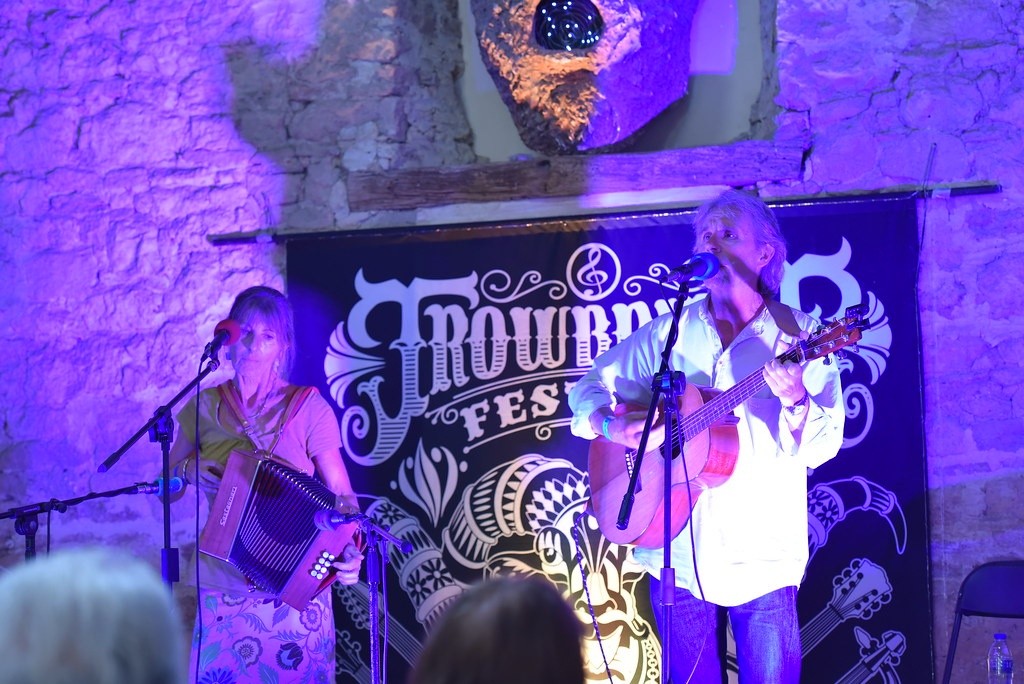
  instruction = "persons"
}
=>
[157,287,364,684]
[406,576,587,684]
[566,187,846,684]
[1,544,184,684]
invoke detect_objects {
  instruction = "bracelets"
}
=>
[174,463,179,478]
[602,416,616,440]
[182,457,191,485]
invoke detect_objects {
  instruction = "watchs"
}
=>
[785,387,809,416]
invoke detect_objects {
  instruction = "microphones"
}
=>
[125,476,184,495]
[660,252,720,283]
[200,319,240,362]
[313,510,362,531]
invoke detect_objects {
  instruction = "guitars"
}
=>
[586,303,872,548]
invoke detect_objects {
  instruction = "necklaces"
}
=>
[247,374,281,419]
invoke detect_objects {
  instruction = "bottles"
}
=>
[987,634,1013,684]
